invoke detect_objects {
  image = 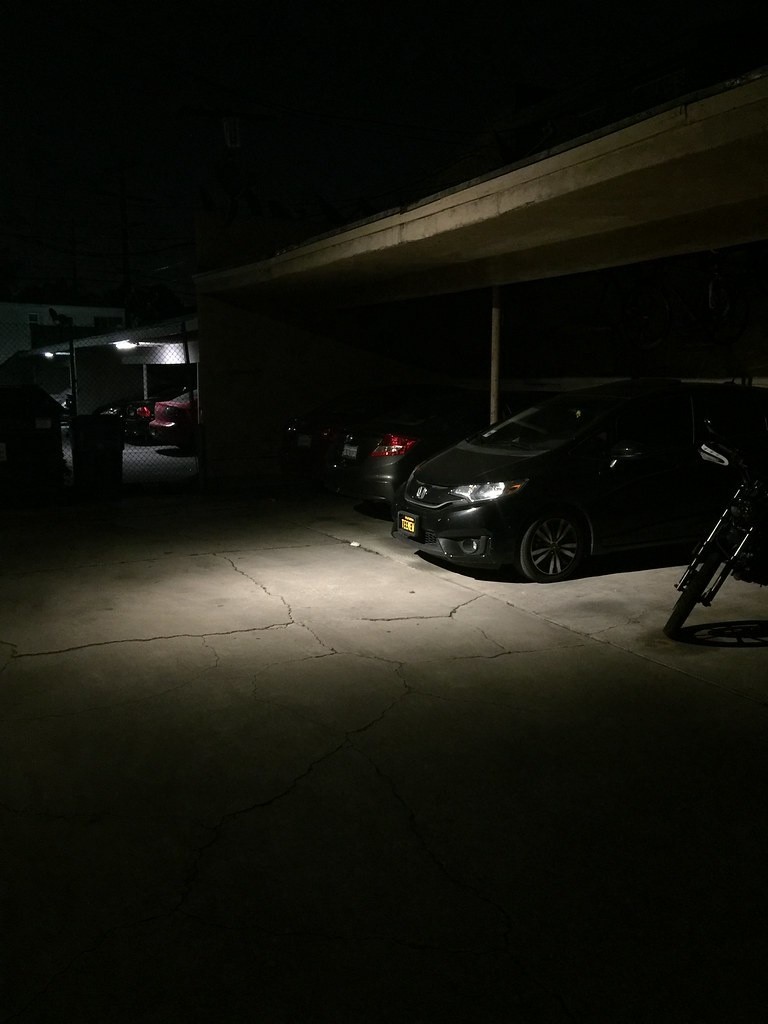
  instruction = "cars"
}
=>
[122,386,193,446]
[279,389,417,488]
[91,392,143,416]
[318,387,555,507]
[143,389,203,454]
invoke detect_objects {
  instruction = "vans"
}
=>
[391,380,768,583]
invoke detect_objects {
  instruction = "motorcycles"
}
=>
[661,411,767,633]
